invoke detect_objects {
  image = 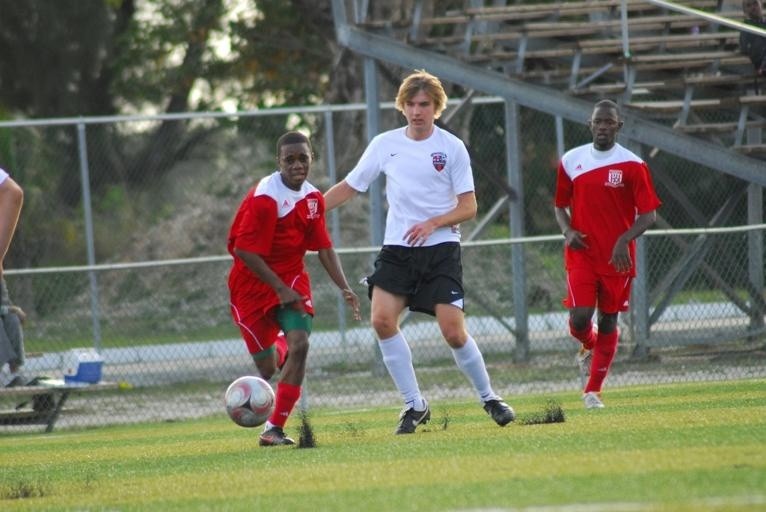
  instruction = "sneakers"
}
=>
[256,425,296,446]
[393,398,432,436]
[575,323,599,377]
[581,392,605,408]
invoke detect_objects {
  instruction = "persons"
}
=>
[0,168,24,278]
[0,279,39,387]
[554,99,662,409]
[227,132,362,446]
[323,68,516,433]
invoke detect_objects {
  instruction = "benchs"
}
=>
[356,0,766,157]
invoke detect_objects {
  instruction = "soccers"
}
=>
[225,376,276,426]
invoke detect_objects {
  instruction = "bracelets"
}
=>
[15,307,21,314]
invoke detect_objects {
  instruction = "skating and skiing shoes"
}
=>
[478,396,515,424]
